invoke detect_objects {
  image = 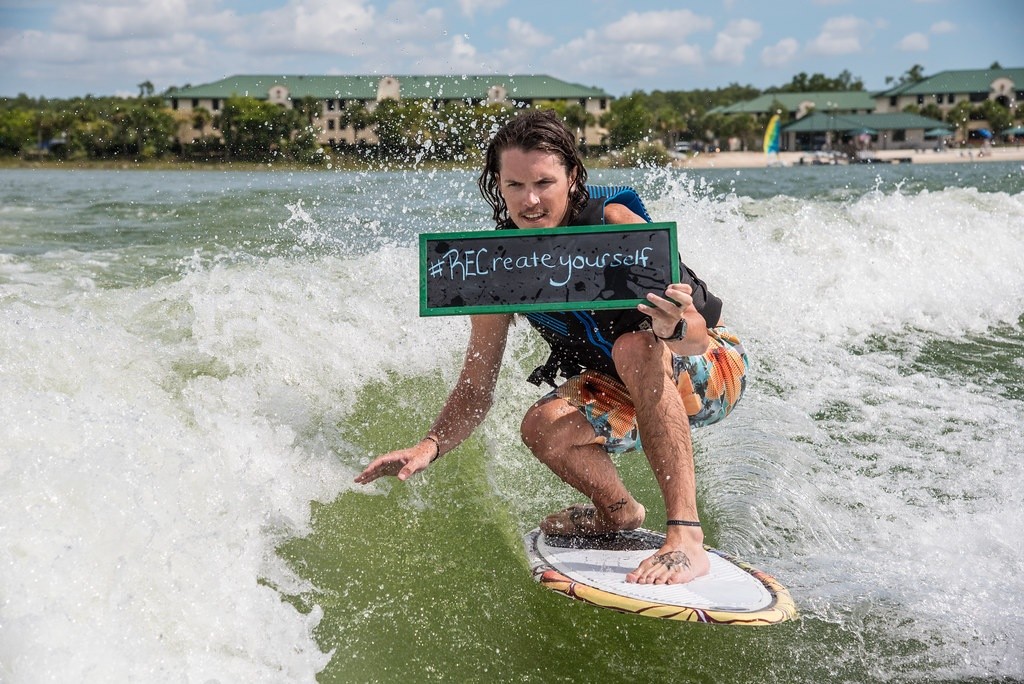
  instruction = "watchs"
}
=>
[652,317,687,343]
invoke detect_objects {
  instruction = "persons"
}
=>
[353,114,748,586]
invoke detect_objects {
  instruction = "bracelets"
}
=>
[424,437,439,462]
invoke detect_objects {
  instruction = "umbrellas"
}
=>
[1001,128,1024,149]
[846,127,879,136]
[925,129,951,147]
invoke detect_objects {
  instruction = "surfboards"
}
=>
[521,522,798,629]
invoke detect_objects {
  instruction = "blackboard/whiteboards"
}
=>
[415,220,681,317]
